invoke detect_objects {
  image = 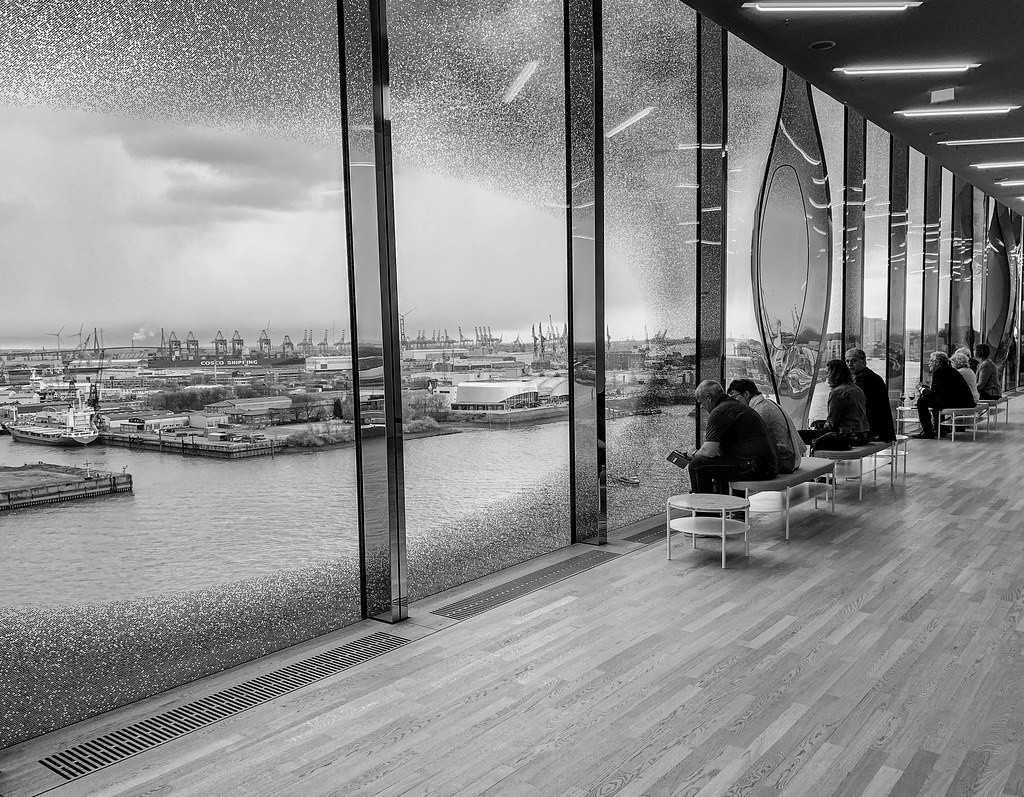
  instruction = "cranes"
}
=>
[160,329,352,359]
[398,308,569,357]
[606,323,672,354]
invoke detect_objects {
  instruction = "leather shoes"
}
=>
[911,424,966,439]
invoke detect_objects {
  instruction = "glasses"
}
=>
[730,391,746,399]
[845,359,864,365]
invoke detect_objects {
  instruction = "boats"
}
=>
[620,475,640,484]
[0,404,16,436]
[4,388,99,447]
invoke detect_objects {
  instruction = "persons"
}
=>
[794,360,870,482]
[811,347,896,485]
[682,380,779,538]
[954,347,978,373]
[950,354,980,431]
[974,344,1001,400]
[713,378,806,517]
[911,351,975,439]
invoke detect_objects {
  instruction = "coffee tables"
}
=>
[667,493,750,568]
[895,407,932,435]
[875,435,909,485]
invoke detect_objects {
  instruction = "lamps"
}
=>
[893,105,1021,116]
[969,162,1023,168]
[937,137,1024,145]
[742,1,924,11]
[994,180,1024,186]
[832,64,982,74]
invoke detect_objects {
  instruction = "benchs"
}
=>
[813,435,894,502]
[728,457,836,539]
[979,395,1008,430]
[938,403,989,441]
